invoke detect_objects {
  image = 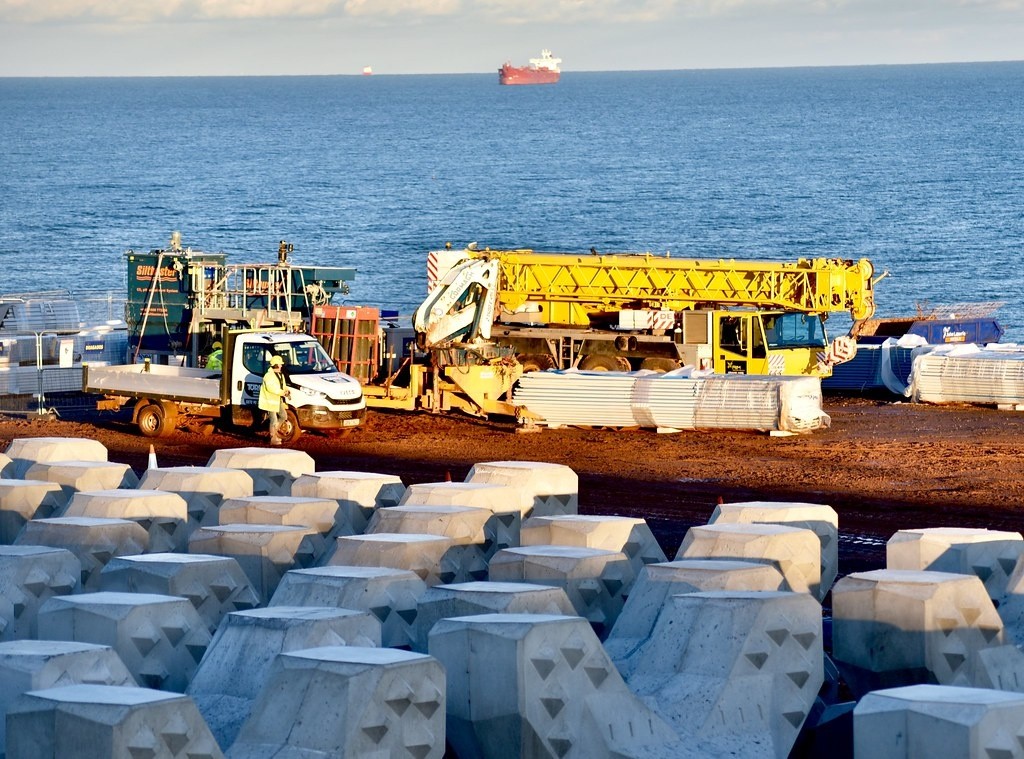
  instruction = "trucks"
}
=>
[82,322,367,448]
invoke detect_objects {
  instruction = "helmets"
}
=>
[270,355,284,366]
[212,341,223,349]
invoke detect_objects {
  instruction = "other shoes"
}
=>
[269,437,282,445]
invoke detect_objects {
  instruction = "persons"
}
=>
[205,342,223,371]
[258,356,290,445]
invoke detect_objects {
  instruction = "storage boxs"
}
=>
[128,252,226,356]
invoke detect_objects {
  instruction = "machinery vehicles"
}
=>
[411,238,883,381]
[359,255,549,436]
[123,229,357,347]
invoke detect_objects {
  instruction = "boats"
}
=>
[498,49,564,85]
[363,65,372,76]
